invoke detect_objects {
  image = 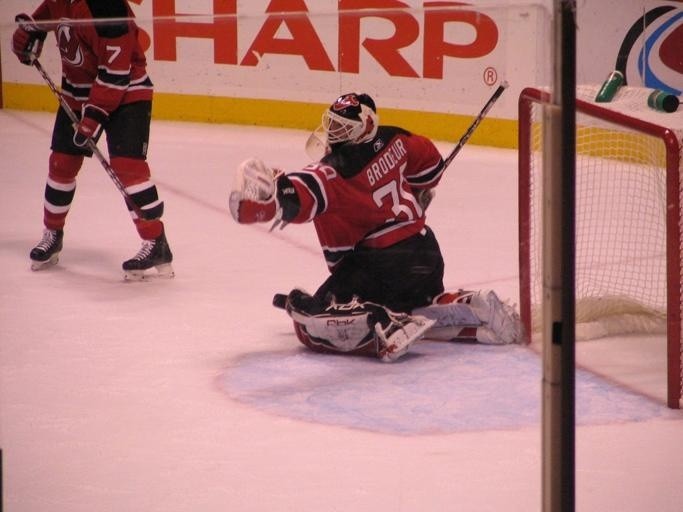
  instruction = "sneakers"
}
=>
[383,312,424,348]
[470,290,517,344]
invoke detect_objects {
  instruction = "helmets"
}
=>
[314,92,379,146]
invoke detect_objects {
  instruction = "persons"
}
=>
[11,0,175,278]
[226,93,522,361]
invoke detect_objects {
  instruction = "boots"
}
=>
[123,223,172,270]
[30,227,65,262]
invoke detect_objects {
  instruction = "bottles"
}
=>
[648,89,679,113]
[595,71,624,103]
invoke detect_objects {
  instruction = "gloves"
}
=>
[10,13,47,67]
[74,103,110,156]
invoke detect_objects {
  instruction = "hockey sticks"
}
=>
[273,79,509,309]
[27,52,163,221]
